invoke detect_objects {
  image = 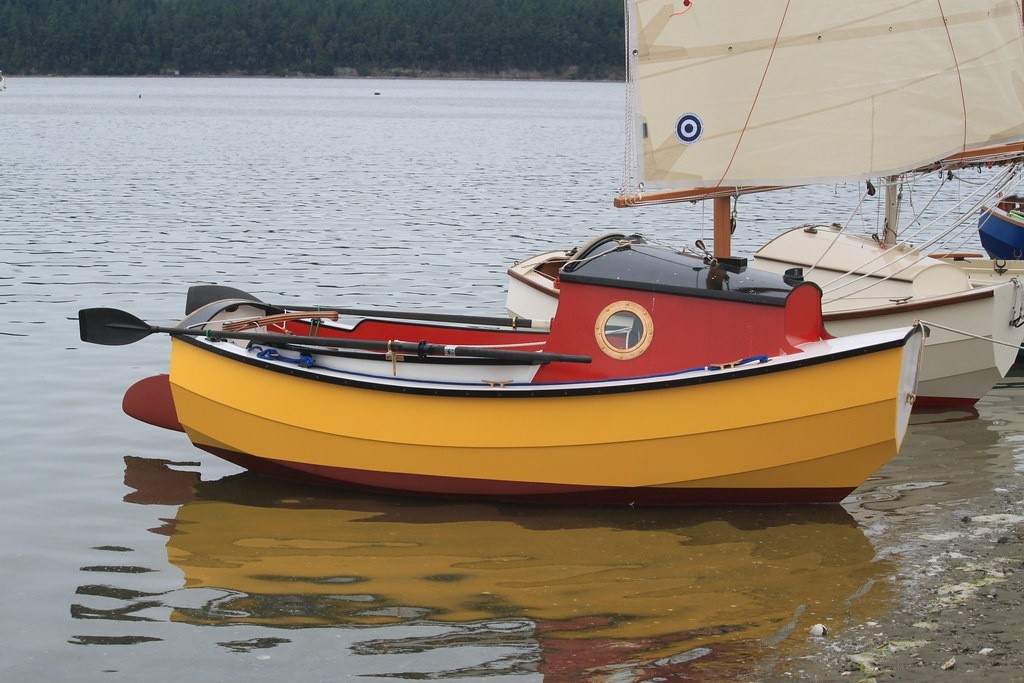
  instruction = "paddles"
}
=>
[78,283,593,365]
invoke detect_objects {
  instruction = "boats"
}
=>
[977,194,1024,261]
[165,471,899,683]
[169,233,930,503]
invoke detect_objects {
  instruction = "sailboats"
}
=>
[505,1,1024,414]
